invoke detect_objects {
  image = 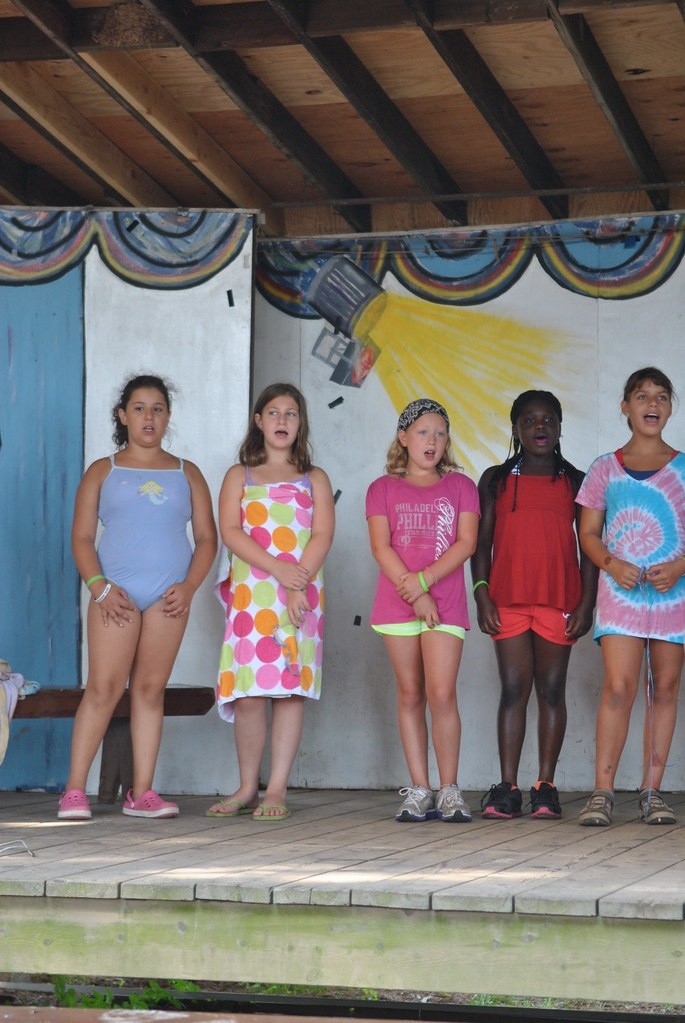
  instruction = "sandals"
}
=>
[123,788,179,819]
[57,789,92,820]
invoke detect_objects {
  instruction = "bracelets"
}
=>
[473,580,488,599]
[93,583,111,603]
[425,566,437,584]
[679,554,685,576]
[418,571,429,592]
[286,587,306,593]
[86,575,104,587]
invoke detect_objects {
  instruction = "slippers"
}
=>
[205,797,255,816]
[252,803,291,821]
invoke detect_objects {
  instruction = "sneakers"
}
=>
[481,781,523,819]
[396,785,438,822]
[530,782,562,819]
[579,787,614,826]
[636,786,677,824]
[435,785,472,822]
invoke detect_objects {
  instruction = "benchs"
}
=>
[11,685,215,805]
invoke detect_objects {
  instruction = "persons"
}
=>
[367,397,480,821]
[471,390,601,818]
[56,374,218,820]
[206,383,336,821]
[575,367,685,826]
